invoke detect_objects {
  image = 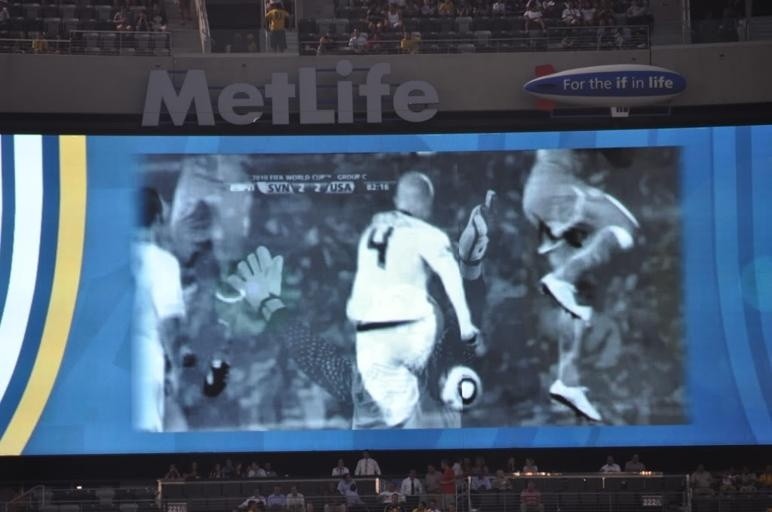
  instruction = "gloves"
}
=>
[227,246,285,322]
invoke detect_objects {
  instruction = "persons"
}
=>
[302,1,655,56]
[0,0,173,56]
[599,453,644,472]
[164,449,545,511]
[691,462,772,496]
[132,147,641,429]
[262,1,291,52]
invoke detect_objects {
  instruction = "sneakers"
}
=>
[548,379,602,422]
[540,273,591,320]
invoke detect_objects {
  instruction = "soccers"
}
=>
[440,366,482,410]
